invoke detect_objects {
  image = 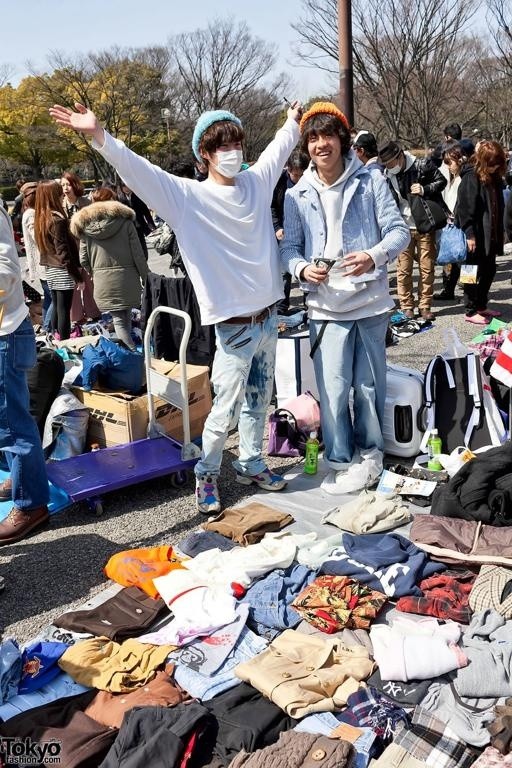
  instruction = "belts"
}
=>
[218,302,278,325]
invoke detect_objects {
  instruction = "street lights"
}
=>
[160,107,174,164]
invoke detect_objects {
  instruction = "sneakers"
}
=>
[193,469,222,517]
[234,466,291,493]
[476,307,502,317]
[333,468,353,487]
[432,289,457,301]
[463,312,490,325]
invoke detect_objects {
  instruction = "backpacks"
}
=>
[418,351,510,462]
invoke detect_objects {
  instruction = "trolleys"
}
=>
[41,300,201,519]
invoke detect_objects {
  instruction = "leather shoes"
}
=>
[417,307,436,320]
[0,478,13,502]
[0,503,51,546]
[401,308,416,320]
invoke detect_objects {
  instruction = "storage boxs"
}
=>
[59,354,214,456]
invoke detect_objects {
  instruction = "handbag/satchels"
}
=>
[405,190,449,236]
[434,214,469,267]
[25,340,66,442]
[428,438,512,529]
[459,263,480,286]
[72,335,145,394]
[40,386,92,461]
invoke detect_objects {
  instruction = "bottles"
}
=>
[303,432,319,475]
[425,429,442,471]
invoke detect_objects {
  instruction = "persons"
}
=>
[0,122,512,354]
[46,91,303,515]
[0,204,51,544]
[279,101,411,471]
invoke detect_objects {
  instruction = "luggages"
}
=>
[346,360,428,460]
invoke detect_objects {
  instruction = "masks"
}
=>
[386,157,403,175]
[205,148,245,180]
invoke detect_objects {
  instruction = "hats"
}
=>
[297,99,350,138]
[19,181,38,198]
[190,108,245,166]
[356,133,377,148]
[376,139,405,166]
